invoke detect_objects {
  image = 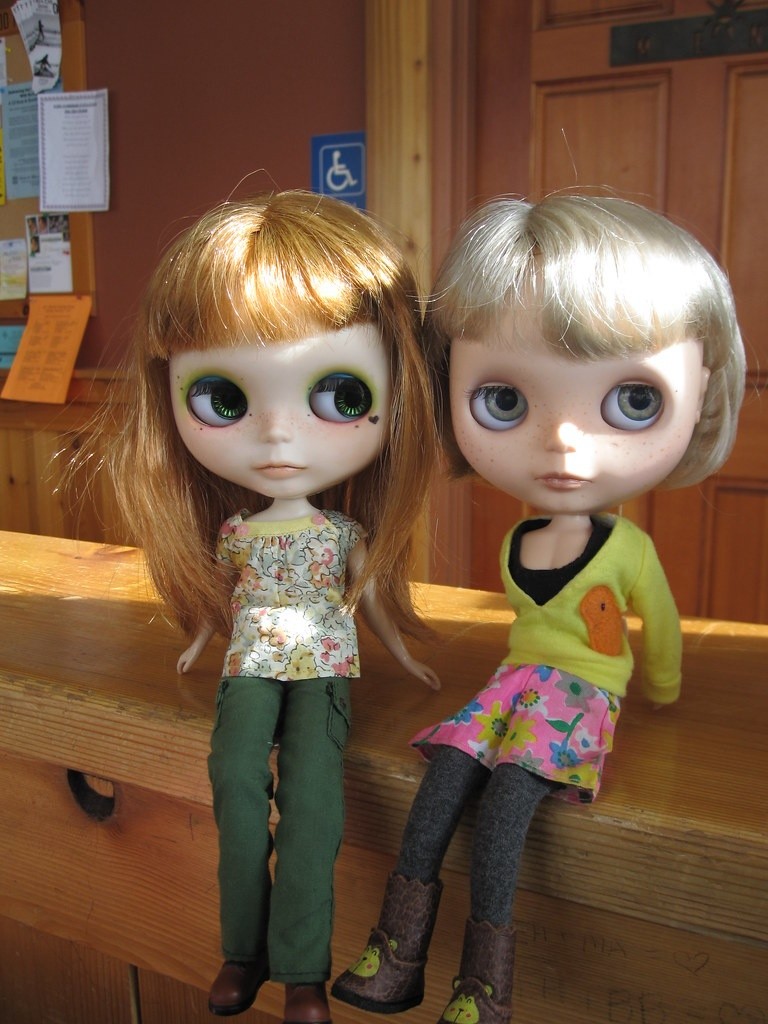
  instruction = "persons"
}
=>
[330,186,748,1023]
[37,188,441,1023]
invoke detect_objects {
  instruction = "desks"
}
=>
[0,531,768,1024]
